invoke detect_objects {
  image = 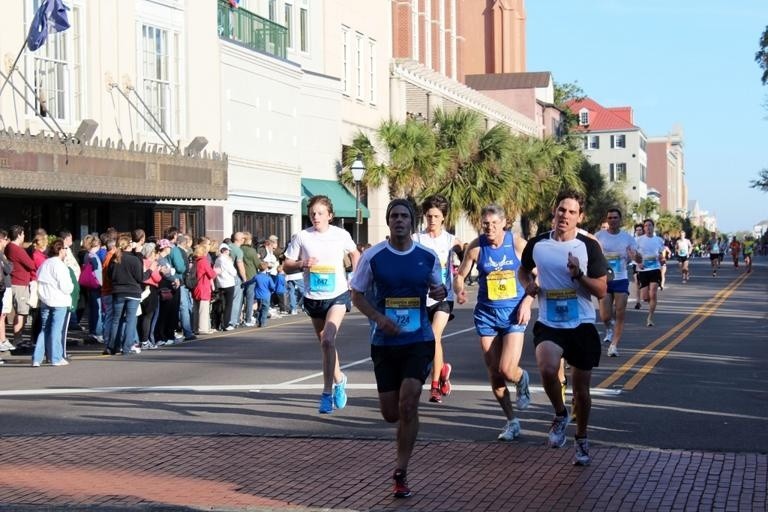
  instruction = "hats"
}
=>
[219,243,231,251]
[269,235,278,240]
[385,200,414,224]
[157,239,174,250]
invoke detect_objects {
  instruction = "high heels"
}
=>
[89,335,104,344]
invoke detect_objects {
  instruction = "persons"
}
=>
[633,219,665,327]
[517,189,608,464]
[28,235,48,346]
[0,229,18,351]
[717,237,724,267]
[551,218,556,230]
[61,266,78,360]
[102,238,117,348]
[283,196,359,413]
[213,243,237,330]
[164,227,197,340]
[222,238,233,246]
[754,240,768,256]
[411,196,465,402]
[193,236,219,246]
[629,223,645,309]
[282,235,306,315]
[730,236,740,267]
[243,231,261,326]
[452,205,538,439]
[80,235,105,344]
[76,235,93,332]
[131,228,145,259]
[60,232,81,279]
[349,199,446,497]
[463,242,474,286]
[96,233,108,264]
[241,261,275,327]
[558,227,603,422]
[157,240,180,345]
[231,232,246,327]
[660,245,671,290]
[141,244,161,350]
[707,232,720,277]
[4,225,35,349]
[275,265,288,315]
[28,228,50,258]
[743,237,753,271]
[594,209,641,356]
[600,217,609,230]
[265,235,280,278]
[675,230,693,284]
[105,236,157,355]
[692,240,708,257]
[31,240,74,366]
[186,246,218,335]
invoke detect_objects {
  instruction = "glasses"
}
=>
[61,248,67,250]
[4,239,8,241]
[481,221,502,228]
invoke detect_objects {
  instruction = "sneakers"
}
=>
[571,399,576,422]
[635,303,641,309]
[333,374,347,408]
[156,341,166,346]
[647,319,655,327]
[682,273,690,283]
[440,364,451,396]
[603,329,613,342]
[319,392,334,413]
[515,371,530,410]
[0,343,8,352]
[392,469,411,498]
[573,434,591,466]
[747,269,753,273]
[52,358,69,366]
[225,325,235,331]
[429,381,442,403]
[32,362,40,367]
[141,340,158,350]
[608,344,619,357]
[713,270,717,276]
[2,340,16,350]
[561,376,568,405]
[498,421,520,440]
[549,411,569,447]
[291,310,297,315]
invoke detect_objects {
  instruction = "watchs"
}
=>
[569,268,583,281]
[440,283,450,298]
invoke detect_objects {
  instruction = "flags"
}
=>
[27,1,71,51]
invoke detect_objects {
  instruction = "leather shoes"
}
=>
[122,350,136,355]
[103,350,115,355]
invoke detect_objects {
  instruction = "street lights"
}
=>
[350,152,367,248]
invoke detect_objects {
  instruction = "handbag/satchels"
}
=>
[78,254,100,288]
